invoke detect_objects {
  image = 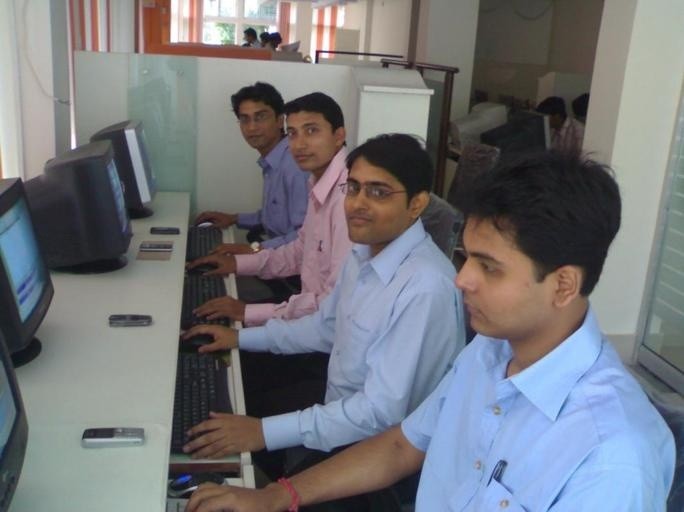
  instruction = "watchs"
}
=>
[250,241,261,254]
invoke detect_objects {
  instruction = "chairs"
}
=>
[424,190,464,264]
[446,141,500,214]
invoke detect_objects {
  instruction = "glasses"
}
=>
[236,112,279,124]
[339,181,417,203]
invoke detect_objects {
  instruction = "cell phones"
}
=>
[139,239,173,252]
[108,313,151,328]
[150,226,181,235]
[82,426,146,448]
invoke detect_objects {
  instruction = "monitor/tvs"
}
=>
[22,139,133,274]
[0,176,55,369]
[0,325,29,512]
[89,118,158,219]
[449,102,551,158]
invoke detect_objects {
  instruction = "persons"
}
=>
[183,150,677,512]
[258,32,270,48]
[262,32,282,53]
[188,92,357,328]
[535,97,585,161]
[179,133,467,461]
[193,81,313,306]
[243,28,261,49]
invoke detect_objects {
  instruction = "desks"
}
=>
[12,190,258,512]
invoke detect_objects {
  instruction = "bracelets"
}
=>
[276,476,300,512]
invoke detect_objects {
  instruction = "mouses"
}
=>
[197,218,215,227]
[194,263,219,273]
[181,333,214,353]
[168,474,228,498]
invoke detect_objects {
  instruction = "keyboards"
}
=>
[181,275,229,330]
[165,499,191,512]
[186,226,223,262]
[171,352,233,456]
